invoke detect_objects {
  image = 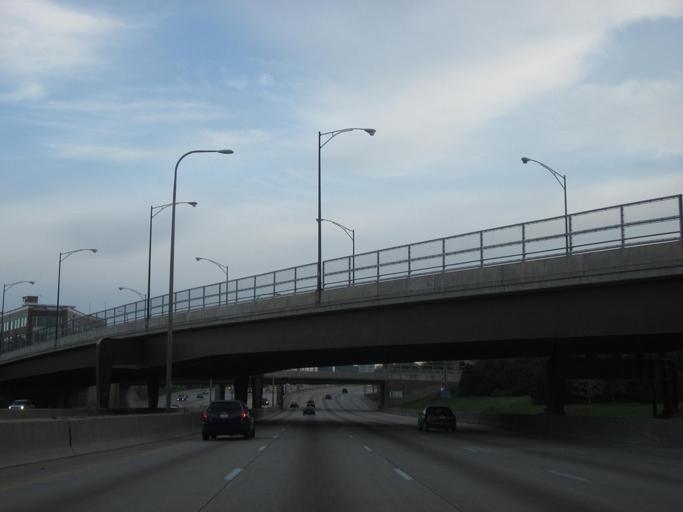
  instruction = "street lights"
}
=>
[164,149,233,412]
[316,127,376,290]
[317,219,355,286]
[195,256,228,305]
[54,248,96,340]
[521,156,568,255]
[146,201,196,319]
[0,281,34,353]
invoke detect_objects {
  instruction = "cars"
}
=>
[176,394,187,401]
[262,398,268,405]
[196,392,203,397]
[290,388,347,416]
[7,398,35,410]
[417,405,456,433]
[203,389,208,394]
[200,399,254,439]
